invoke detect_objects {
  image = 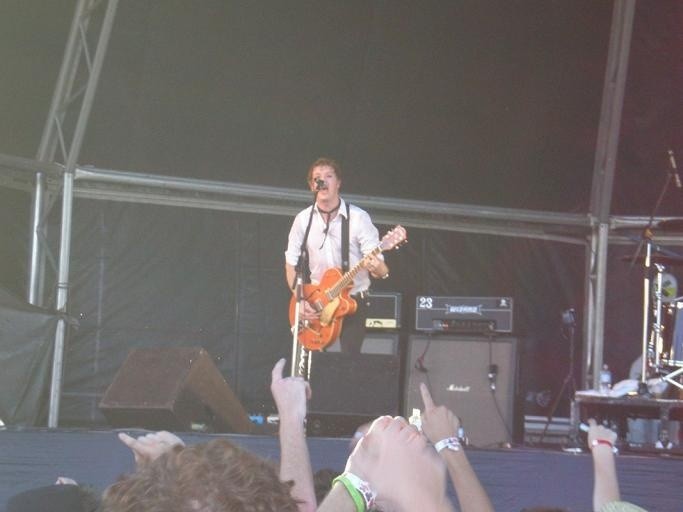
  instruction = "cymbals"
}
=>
[658,219,683,232]
[619,253,682,264]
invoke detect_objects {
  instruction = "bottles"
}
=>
[599,364,612,396]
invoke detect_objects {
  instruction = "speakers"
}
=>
[305,351,402,438]
[99,345,259,435]
[403,334,528,450]
[325,332,402,354]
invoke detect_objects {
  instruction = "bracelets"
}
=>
[379,273,389,281]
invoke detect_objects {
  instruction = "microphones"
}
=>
[667,149,682,188]
[314,178,328,190]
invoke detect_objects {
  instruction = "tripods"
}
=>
[536,325,580,443]
[618,171,674,399]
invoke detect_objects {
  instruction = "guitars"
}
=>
[289,225,408,351]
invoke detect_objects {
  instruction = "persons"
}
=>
[284,158,389,442]
[3,358,649,512]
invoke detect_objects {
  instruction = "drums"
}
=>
[659,301,682,369]
